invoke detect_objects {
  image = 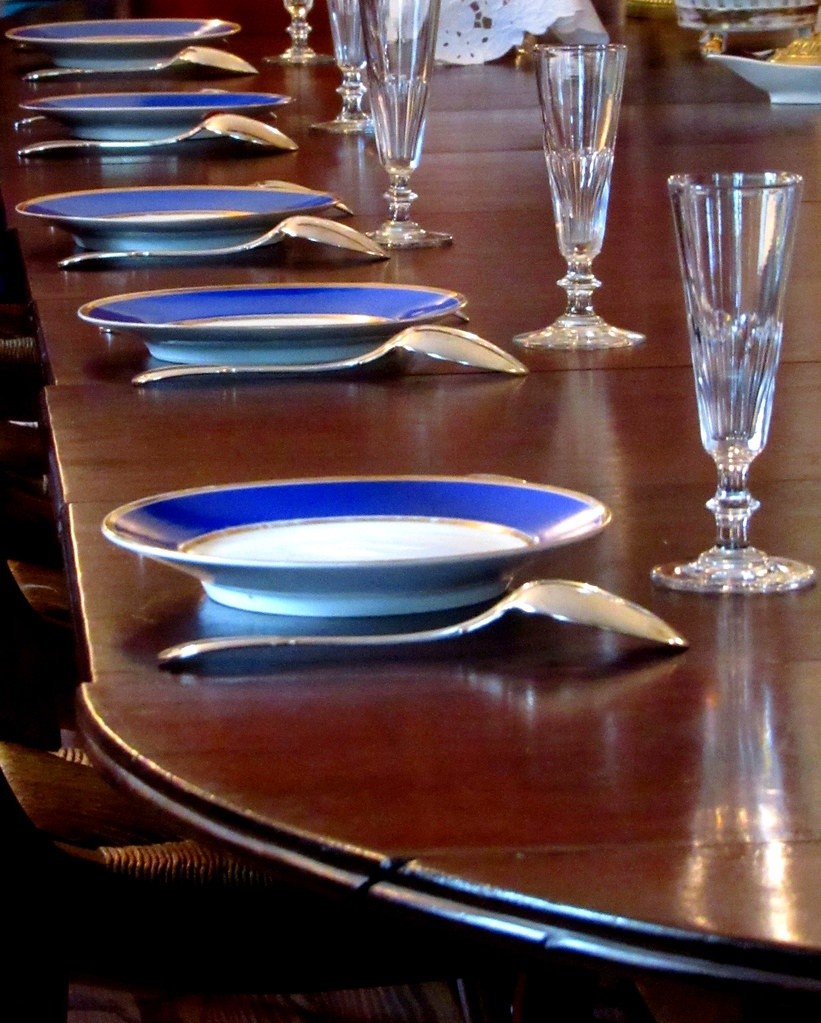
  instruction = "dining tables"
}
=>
[0,1,821,990]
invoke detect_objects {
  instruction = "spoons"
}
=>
[24,46,259,79]
[57,214,391,273]
[252,178,352,214]
[160,580,689,662]
[132,325,528,384]
[17,113,298,156]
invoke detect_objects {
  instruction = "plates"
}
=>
[709,53,821,104]
[13,88,292,128]
[15,188,339,254]
[5,18,241,71]
[100,475,612,619]
[78,283,467,369]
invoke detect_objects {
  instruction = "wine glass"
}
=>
[263,0,336,65]
[651,172,816,598]
[513,43,645,350]
[311,0,375,136]
[359,0,453,247]
